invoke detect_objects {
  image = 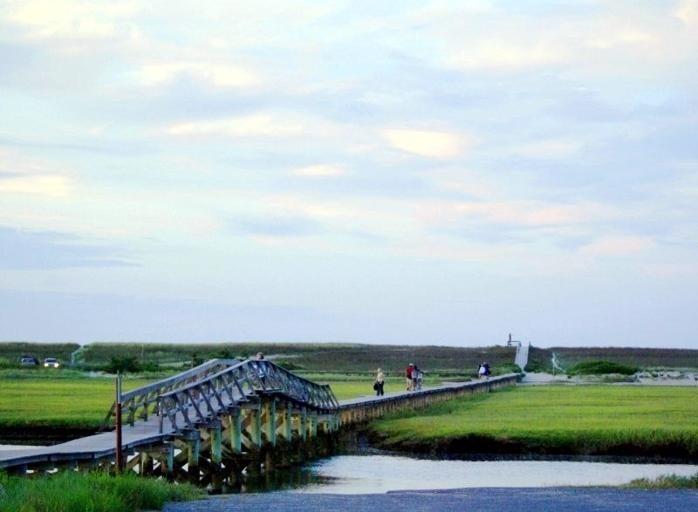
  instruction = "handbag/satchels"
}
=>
[374,383,378,390]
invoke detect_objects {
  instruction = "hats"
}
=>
[409,364,413,367]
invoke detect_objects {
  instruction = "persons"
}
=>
[477,361,492,381]
[375,368,384,397]
[252,352,266,389]
[405,362,423,392]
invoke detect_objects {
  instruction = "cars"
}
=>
[43,357,59,368]
[19,356,35,367]
